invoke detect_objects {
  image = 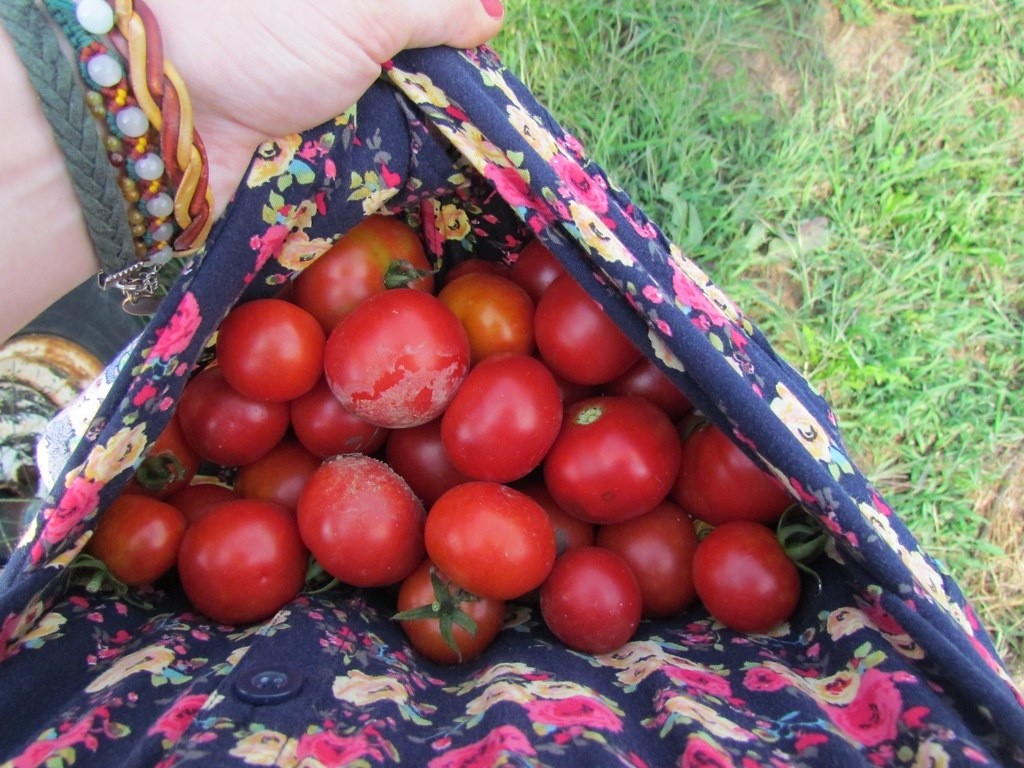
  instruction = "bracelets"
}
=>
[0,0,213,317]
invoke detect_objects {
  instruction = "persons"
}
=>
[0,0,1024,768]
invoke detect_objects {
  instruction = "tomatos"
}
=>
[93,215,827,661]
